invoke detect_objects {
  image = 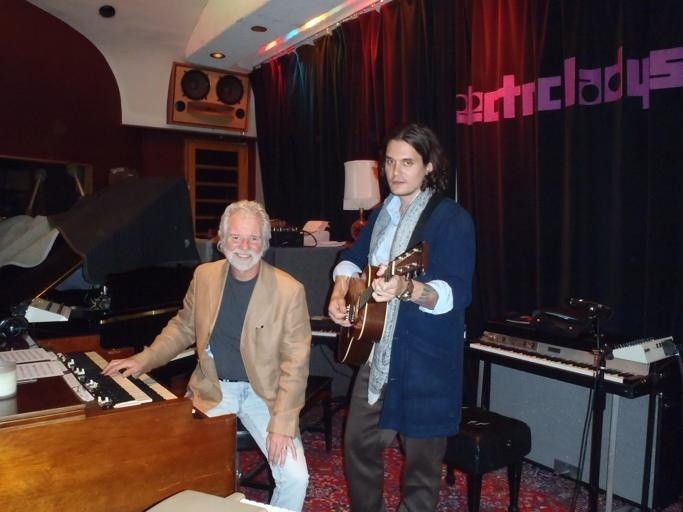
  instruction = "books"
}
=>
[0,348,64,381]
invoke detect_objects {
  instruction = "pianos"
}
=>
[1,177,236,512]
[469,312,677,399]
[310,316,340,344]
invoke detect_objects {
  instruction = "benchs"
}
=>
[234,375,334,497]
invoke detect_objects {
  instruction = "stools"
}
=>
[440,404,533,512]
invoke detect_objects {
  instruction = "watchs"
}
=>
[397,280,414,302]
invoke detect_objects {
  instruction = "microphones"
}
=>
[26,168,47,215]
[570,296,613,315]
[66,164,86,198]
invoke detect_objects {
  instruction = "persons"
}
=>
[102,199,312,512]
[327,122,477,511]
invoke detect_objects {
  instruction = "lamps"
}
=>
[342,155,384,242]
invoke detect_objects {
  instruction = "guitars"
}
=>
[336,242,429,366]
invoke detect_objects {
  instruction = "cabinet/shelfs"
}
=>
[183,135,252,241]
